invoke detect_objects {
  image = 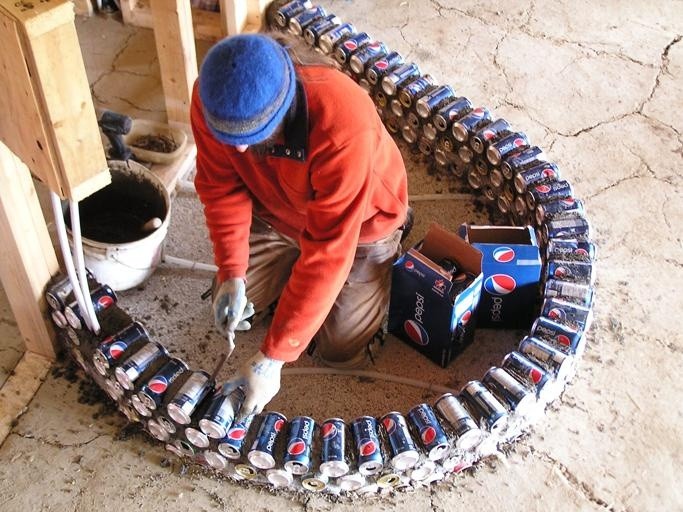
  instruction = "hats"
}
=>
[198,31,297,145]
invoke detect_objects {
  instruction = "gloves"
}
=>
[210,278,256,344]
[220,349,286,425]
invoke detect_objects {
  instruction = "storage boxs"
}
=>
[457,221,546,330]
[384,220,486,370]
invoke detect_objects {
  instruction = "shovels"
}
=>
[209,296,248,384]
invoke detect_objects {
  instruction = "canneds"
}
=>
[44,1,598,496]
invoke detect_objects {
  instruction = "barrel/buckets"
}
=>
[59,159,171,290]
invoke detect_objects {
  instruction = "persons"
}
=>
[189,33,409,426]
